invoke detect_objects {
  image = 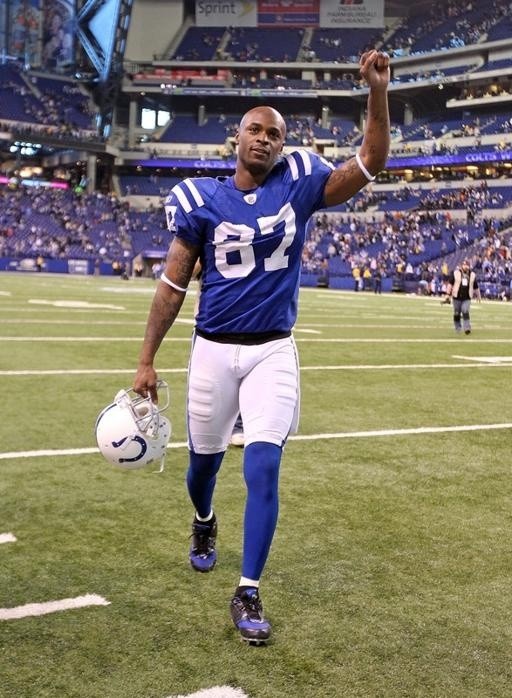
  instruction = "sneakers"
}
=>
[189,521,217,572]
[230,589,272,645]
[231,415,245,446]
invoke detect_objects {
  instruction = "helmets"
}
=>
[95,380,172,474]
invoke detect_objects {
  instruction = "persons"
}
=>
[133,49,395,647]
[444,258,483,335]
[1,0,512,303]
